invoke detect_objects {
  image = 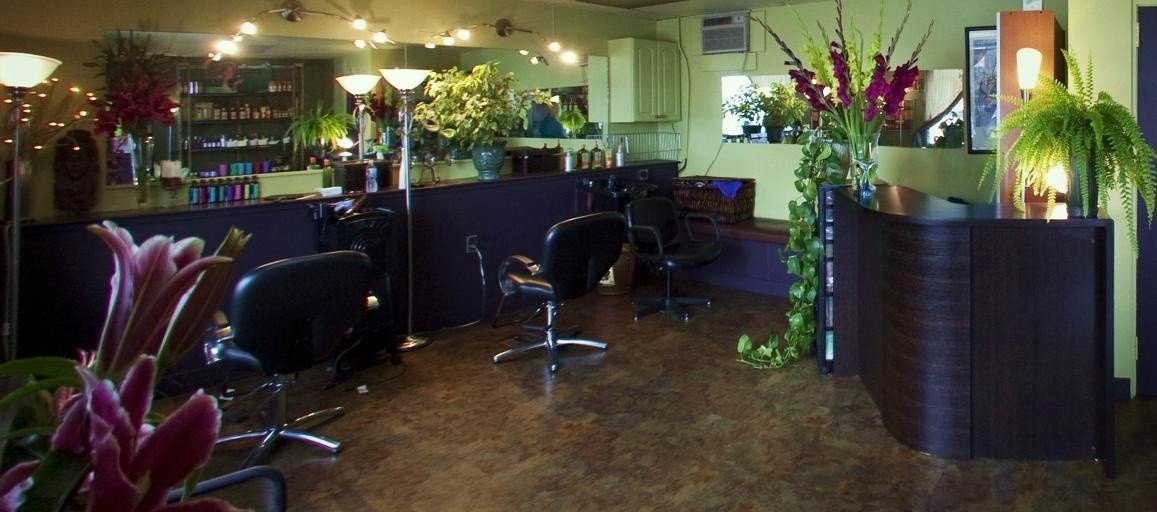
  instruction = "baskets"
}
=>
[672,176,755,224]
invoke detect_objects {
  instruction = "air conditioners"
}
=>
[700,10,750,53]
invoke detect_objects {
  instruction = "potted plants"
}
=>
[559,104,586,139]
[934,111,965,148]
[282,96,357,159]
[413,60,560,180]
[738,118,852,368]
[722,81,809,143]
[978,48,1157,259]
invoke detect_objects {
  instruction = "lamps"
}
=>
[380,69,430,352]
[335,74,384,159]
[0,51,63,359]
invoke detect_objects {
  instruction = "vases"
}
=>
[848,134,881,197]
[130,122,158,184]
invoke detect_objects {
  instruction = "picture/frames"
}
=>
[965,25,997,155]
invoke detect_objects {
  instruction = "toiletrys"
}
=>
[169,80,296,148]
[186,174,263,204]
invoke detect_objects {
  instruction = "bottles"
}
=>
[548,141,625,173]
[136,166,151,204]
[307,155,321,170]
[366,160,378,193]
[220,134,226,146]
[322,158,330,170]
[263,157,270,173]
[188,174,259,203]
[213,100,251,122]
[183,81,199,94]
[276,79,293,92]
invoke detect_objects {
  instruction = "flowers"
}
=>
[744,0,933,181]
[82,29,184,135]
[0,222,253,512]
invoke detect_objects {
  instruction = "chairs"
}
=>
[493,212,627,374]
[625,198,723,322]
[201,249,372,472]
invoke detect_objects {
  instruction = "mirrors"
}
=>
[105,29,609,189]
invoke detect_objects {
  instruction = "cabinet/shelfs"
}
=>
[608,37,682,123]
[171,63,305,176]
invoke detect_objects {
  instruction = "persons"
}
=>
[220,64,244,94]
[539,102,567,138]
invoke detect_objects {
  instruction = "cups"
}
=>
[218,161,262,176]
